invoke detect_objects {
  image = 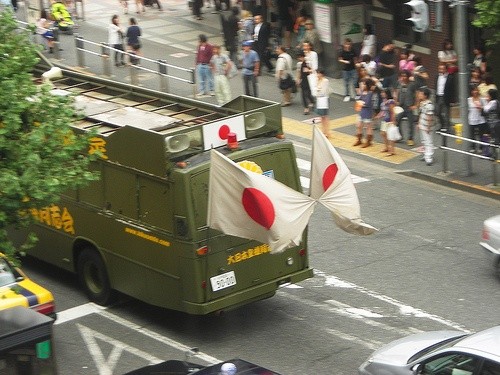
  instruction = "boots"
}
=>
[353,134,363,146]
[361,134,373,148]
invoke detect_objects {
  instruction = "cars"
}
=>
[120,358,283,375]
[479,214,500,274]
[0,253,57,322]
[356,325,500,375]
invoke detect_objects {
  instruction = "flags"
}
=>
[207,149,316,252]
[308,126,380,236]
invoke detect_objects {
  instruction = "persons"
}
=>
[479,62,491,82]
[480,72,498,100]
[338,24,428,102]
[469,67,482,94]
[417,89,435,165]
[435,64,456,133]
[108,0,163,67]
[353,79,372,148]
[477,89,499,158]
[375,70,415,156]
[295,53,314,115]
[467,86,489,154]
[438,39,456,65]
[471,45,484,69]
[267,0,324,107]
[9,0,76,52]
[314,68,329,138]
[190,0,274,104]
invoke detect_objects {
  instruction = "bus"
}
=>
[4,49,316,318]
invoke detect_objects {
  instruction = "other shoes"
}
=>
[380,148,388,153]
[407,140,414,146]
[386,152,395,157]
[304,109,310,115]
[427,162,433,166]
[420,155,426,161]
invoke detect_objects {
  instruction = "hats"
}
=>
[242,41,251,46]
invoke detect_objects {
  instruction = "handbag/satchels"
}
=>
[386,123,402,142]
[279,74,294,91]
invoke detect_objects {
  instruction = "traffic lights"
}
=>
[403,0,430,33]
